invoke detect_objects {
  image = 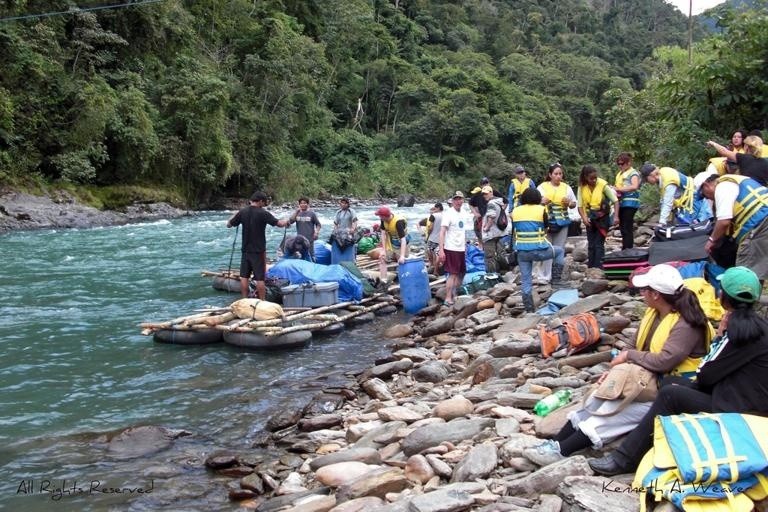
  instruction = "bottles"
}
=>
[611,350,618,359]
[534,389,571,415]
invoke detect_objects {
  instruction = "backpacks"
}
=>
[537,312,603,360]
[494,202,508,231]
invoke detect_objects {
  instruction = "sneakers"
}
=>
[522,438,554,457]
[443,299,454,308]
[525,440,572,467]
[372,281,390,293]
[587,450,638,476]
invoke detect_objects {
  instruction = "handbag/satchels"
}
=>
[709,234,739,270]
[582,362,659,417]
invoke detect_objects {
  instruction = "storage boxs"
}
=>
[281,281,340,310]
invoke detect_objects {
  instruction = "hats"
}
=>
[341,196,351,208]
[640,161,656,182]
[715,265,762,303]
[447,165,526,205]
[693,171,718,200]
[632,263,685,295]
[375,207,391,217]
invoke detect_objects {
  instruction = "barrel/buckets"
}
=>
[330,239,358,264]
[396,258,431,313]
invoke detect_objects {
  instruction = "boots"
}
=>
[521,293,536,313]
[549,263,571,289]
